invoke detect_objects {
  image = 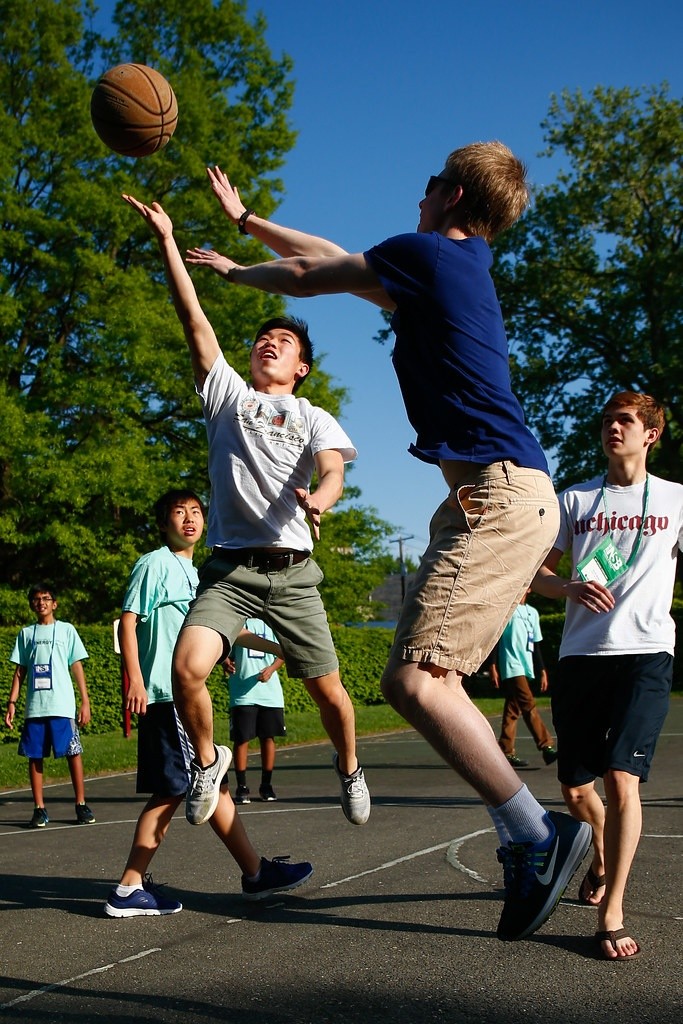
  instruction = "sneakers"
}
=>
[105,873,182,917]
[543,746,557,765]
[496,810,594,942]
[30,805,49,827]
[241,856,313,900]
[507,756,529,768]
[332,752,371,825]
[75,802,96,824]
[185,744,233,824]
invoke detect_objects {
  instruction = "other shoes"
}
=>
[259,784,277,801]
[235,785,251,804]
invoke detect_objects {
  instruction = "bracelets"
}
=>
[238,209,257,235]
[7,701,16,708]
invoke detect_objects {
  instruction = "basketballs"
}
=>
[90,63,180,159]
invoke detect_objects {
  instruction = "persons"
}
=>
[5,582,97,827]
[530,391,683,964]
[120,187,374,825]
[183,138,594,941]
[489,583,558,768]
[221,617,288,804]
[103,487,314,920]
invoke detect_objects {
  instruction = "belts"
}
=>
[213,546,309,571]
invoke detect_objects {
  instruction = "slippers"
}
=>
[596,928,643,961]
[578,863,606,906]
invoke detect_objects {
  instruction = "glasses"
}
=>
[424,175,456,196]
[32,596,53,603]
